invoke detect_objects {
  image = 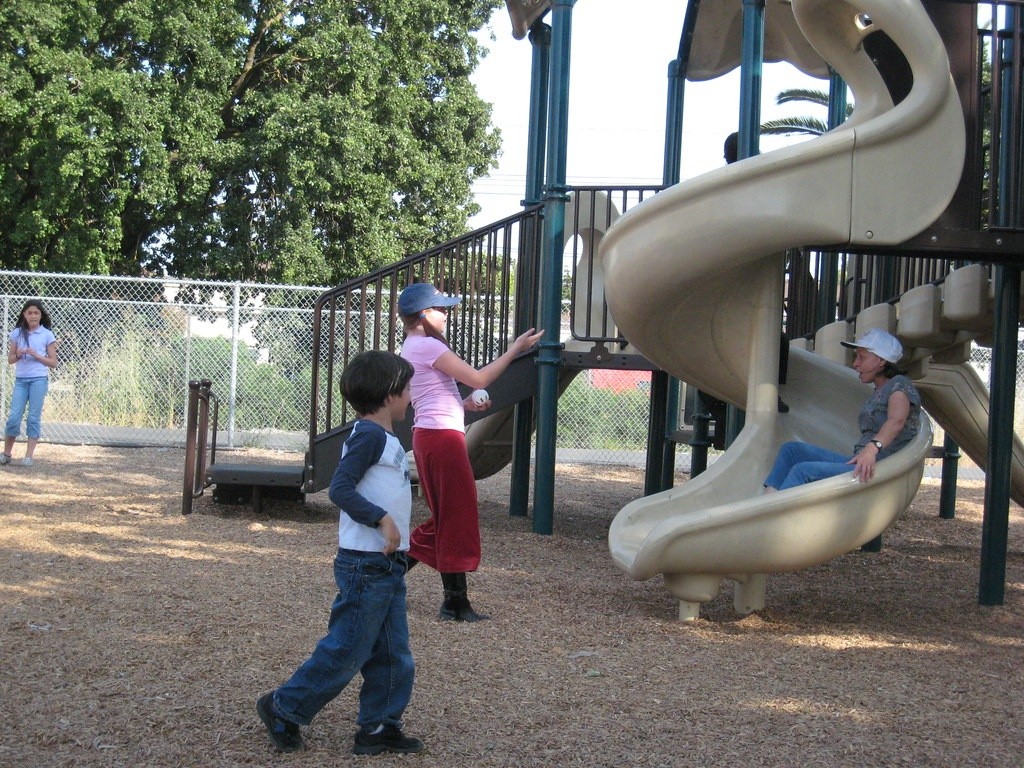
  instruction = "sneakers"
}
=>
[354,727,422,755]
[256,691,302,752]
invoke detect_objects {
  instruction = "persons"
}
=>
[398,283,546,622]
[763,328,921,495]
[0,300,56,465]
[256,350,424,755]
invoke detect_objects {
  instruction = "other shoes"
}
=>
[22,456,32,465]
[439,590,489,623]
[0,454,12,464]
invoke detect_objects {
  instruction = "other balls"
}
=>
[472,390,489,406]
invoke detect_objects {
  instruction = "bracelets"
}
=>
[16,355,21,359]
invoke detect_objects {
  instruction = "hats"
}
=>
[840,330,904,363]
[398,283,461,317]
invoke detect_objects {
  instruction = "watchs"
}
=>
[870,440,882,453]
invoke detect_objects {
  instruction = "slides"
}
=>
[912,362,1024,508]
[596,103,971,583]
[408,341,596,485]
